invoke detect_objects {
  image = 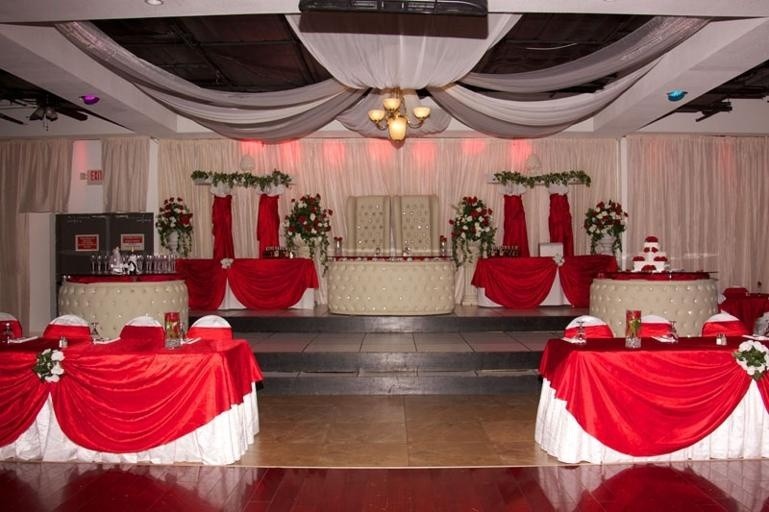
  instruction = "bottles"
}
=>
[492,244,520,256]
[264,246,291,257]
[716,332,728,346]
[179,322,187,339]
[163,311,181,351]
[335,240,342,258]
[110,245,123,275]
[440,239,449,258]
[623,309,643,348]
[58,336,68,349]
[127,245,137,274]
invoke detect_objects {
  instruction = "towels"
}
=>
[8,336,38,345]
[742,333,769,342]
[560,336,587,345]
[93,336,120,345]
[184,336,201,345]
[652,334,678,343]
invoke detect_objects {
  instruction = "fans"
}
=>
[24,87,89,124]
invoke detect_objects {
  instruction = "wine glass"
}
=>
[574,319,588,346]
[666,320,680,344]
[0,321,17,342]
[89,252,177,274]
[88,321,103,346]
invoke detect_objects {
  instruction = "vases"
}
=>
[598,230,615,259]
[164,310,180,349]
[625,307,642,348]
[165,232,187,255]
[297,233,320,257]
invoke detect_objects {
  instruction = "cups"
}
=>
[755,316,768,336]
[663,263,674,280]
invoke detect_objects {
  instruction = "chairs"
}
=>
[190,316,234,338]
[539,241,565,258]
[0,312,22,338]
[344,194,391,254]
[702,311,754,337]
[40,311,92,338]
[115,312,165,340]
[627,313,678,337]
[391,194,441,257]
[560,311,614,336]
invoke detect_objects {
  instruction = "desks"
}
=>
[325,252,458,316]
[173,257,316,309]
[470,257,620,310]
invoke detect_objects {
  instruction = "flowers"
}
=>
[625,309,641,338]
[584,200,630,237]
[730,339,768,376]
[30,344,67,383]
[165,312,179,340]
[153,197,194,240]
[285,194,331,244]
[449,197,496,240]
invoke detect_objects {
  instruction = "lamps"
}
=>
[369,87,433,145]
[35,104,58,121]
